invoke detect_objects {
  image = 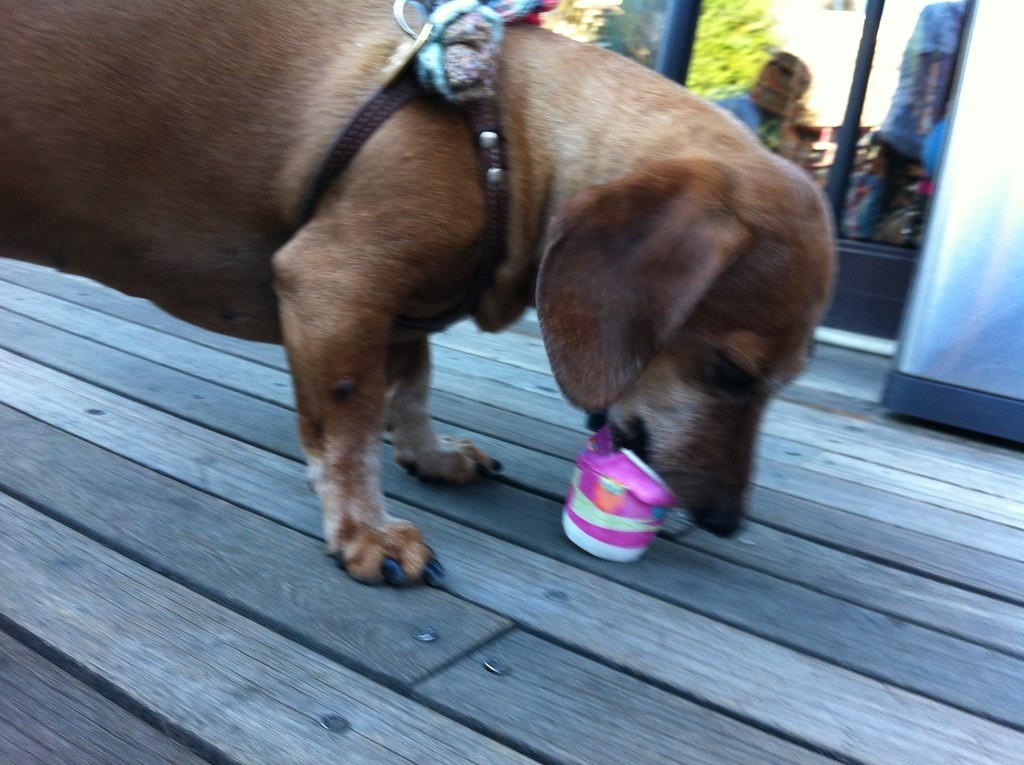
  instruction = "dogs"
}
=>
[0,0,837,588]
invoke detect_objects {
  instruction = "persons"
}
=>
[716,52,811,143]
[844,0,973,252]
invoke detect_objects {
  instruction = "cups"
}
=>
[563,423,681,564]
